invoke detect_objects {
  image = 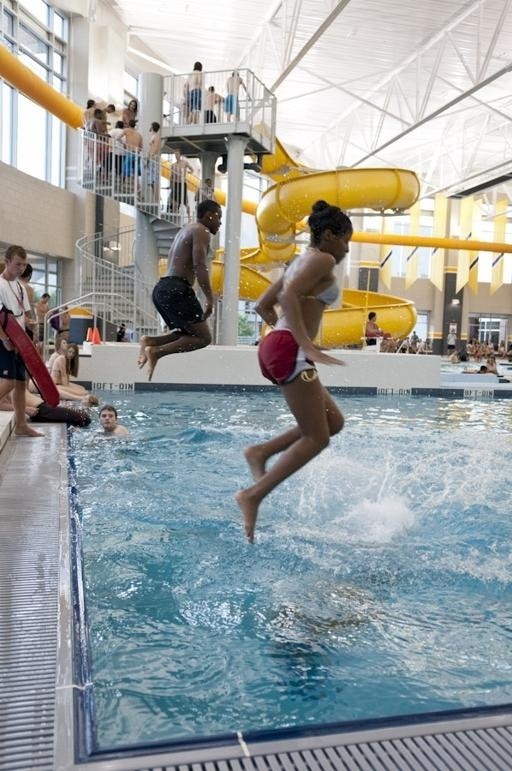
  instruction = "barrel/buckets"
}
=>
[65,313,95,344]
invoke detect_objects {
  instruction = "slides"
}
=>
[158,120,420,348]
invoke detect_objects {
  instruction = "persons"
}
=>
[0,245,129,439]
[235,198,353,546]
[77,98,217,223]
[136,201,223,382]
[366,311,512,375]
[180,61,254,127]
